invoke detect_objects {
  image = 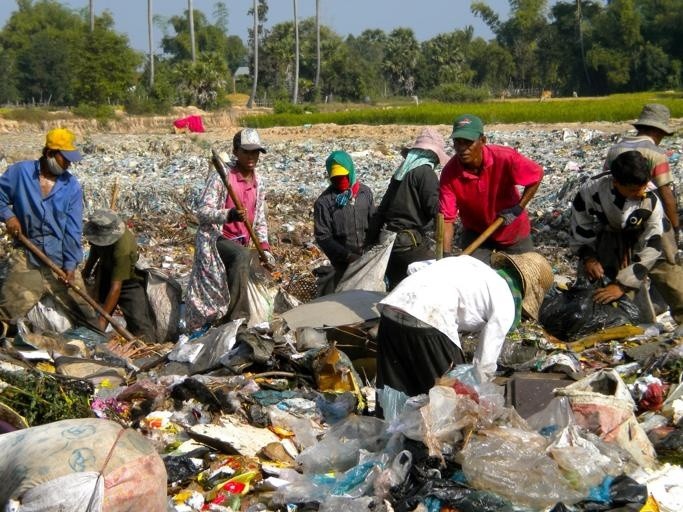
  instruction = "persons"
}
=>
[600,102,683,314]
[374,251,554,418]
[0,129,99,344]
[437,114,545,265]
[369,127,451,291]
[567,150,683,323]
[185,126,275,334]
[314,150,378,276]
[82,206,156,347]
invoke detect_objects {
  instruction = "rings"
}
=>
[15,230,19,233]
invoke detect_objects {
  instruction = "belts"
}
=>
[382,306,434,329]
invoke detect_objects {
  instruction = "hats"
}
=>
[82,209,125,247]
[231,127,265,155]
[45,127,82,163]
[329,162,349,180]
[399,127,451,168]
[448,115,485,143]
[487,249,553,322]
[630,102,675,138]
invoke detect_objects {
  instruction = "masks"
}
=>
[43,156,66,176]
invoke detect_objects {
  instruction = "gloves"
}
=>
[497,204,523,227]
